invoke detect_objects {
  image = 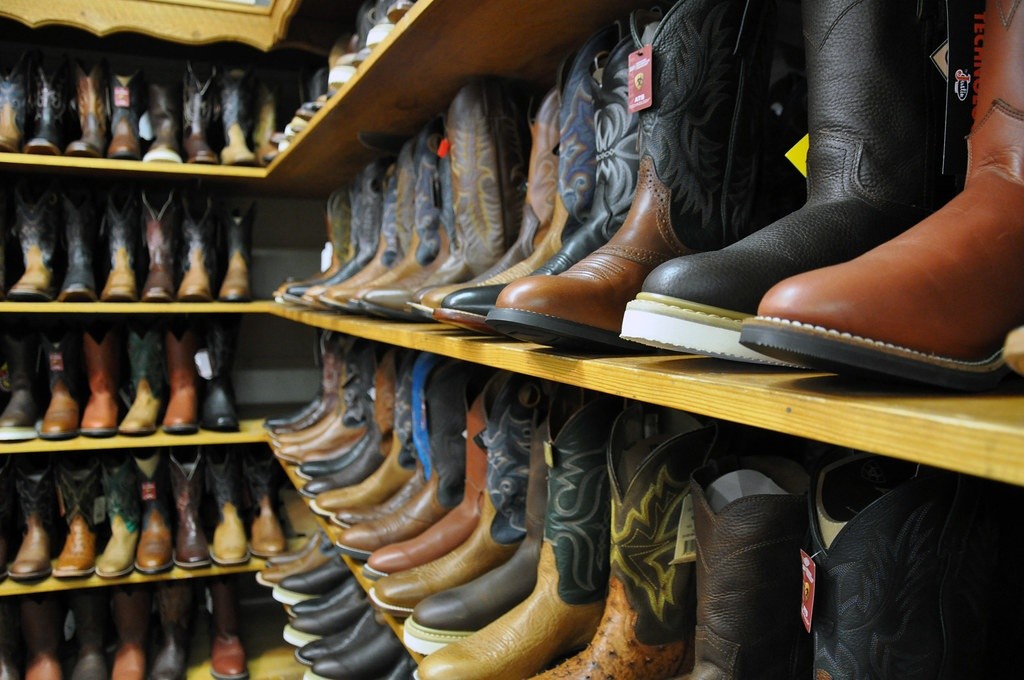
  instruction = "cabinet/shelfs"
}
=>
[0,0,1024,680]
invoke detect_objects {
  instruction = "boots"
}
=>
[0,0,1024,680]
